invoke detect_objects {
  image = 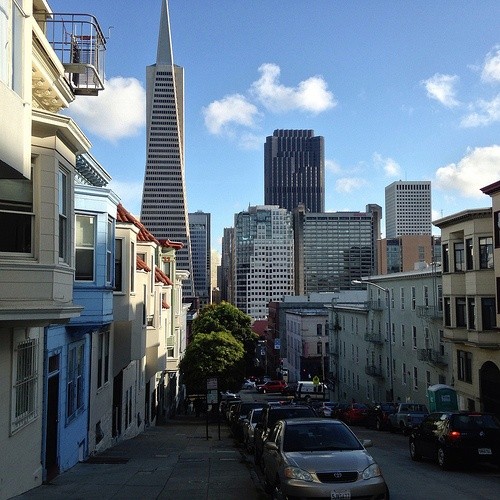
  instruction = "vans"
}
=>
[294,380,330,399]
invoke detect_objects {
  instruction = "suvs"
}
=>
[254,400,324,454]
[366,402,400,431]
[256,379,289,393]
[385,401,430,436]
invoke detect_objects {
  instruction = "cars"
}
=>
[255,417,389,500]
[410,410,500,473]
[315,401,339,418]
[219,393,294,454]
[340,402,371,426]
[240,379,256,390]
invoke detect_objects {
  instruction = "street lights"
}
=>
[351,280,393,403]
